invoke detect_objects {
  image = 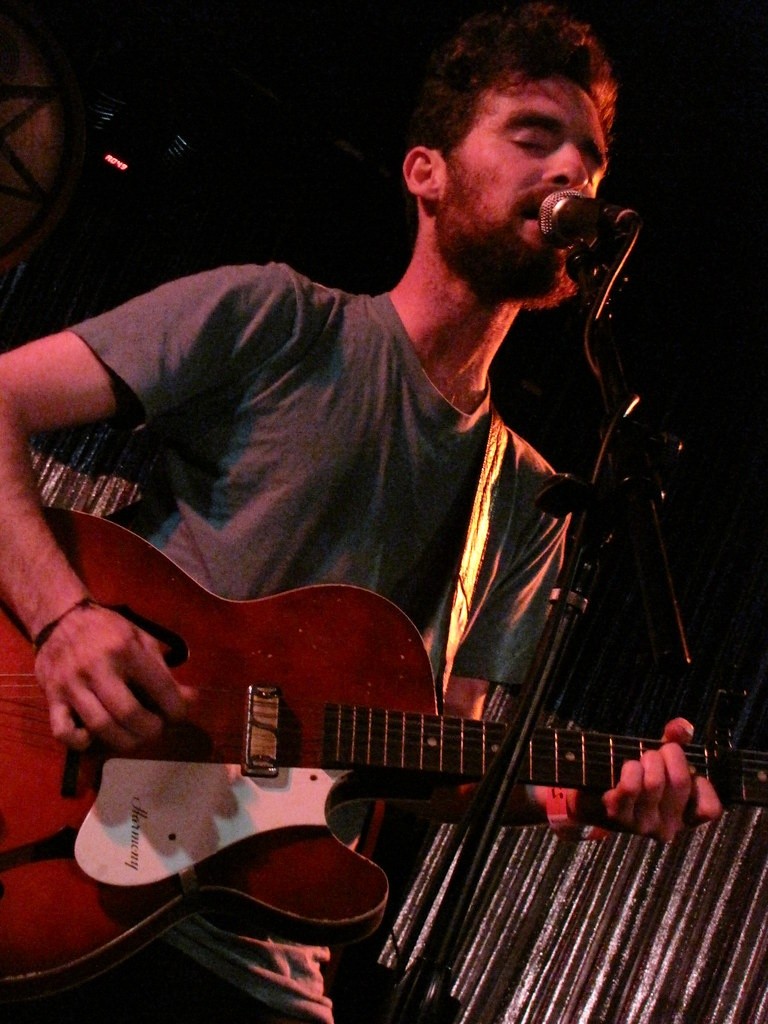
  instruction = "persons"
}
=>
[0,5,721,1024]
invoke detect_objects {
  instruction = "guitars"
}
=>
[1,505,767,1006]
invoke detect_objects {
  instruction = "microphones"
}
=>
[538,190,640,249]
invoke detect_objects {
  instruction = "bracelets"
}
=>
[544,784,606,842]
[32,595,96,650]
